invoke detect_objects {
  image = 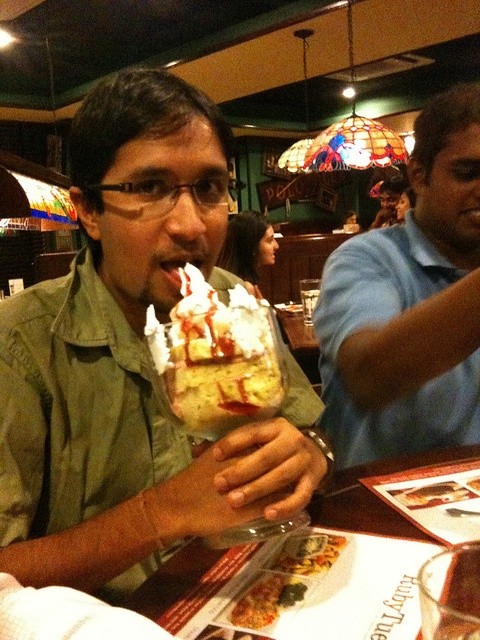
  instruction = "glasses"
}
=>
[81,169,229,207]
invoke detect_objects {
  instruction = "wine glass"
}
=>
[146,301,313,548]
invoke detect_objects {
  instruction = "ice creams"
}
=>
[144,262,287,433]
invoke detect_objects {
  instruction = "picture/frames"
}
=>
[261,147,301,182]
[313,181,340,213]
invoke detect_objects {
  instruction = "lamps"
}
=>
[301,1,410,176]
[273,28,315,173]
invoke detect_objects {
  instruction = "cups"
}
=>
[299,281,321,326]
[417,544,480,636]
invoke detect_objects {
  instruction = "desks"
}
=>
[116,442,480,639]
[272,300,329,350]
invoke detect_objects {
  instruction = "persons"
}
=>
[395,187,416,221]
[272,224,283,238]
[332,210,363,234]
[367,182,405,229]
[217,211,279,297]
[0,67,337,592]
[315,84,479,466]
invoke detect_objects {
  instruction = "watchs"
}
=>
[299,428,336,479]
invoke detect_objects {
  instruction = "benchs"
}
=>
[260,231,360,307]
[33,249,79,285]
